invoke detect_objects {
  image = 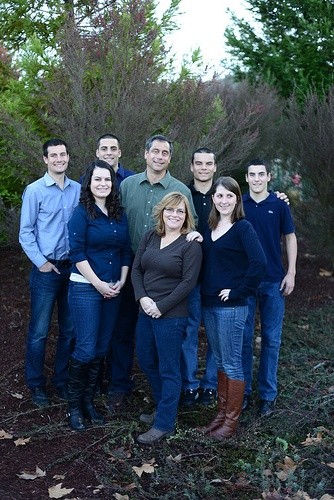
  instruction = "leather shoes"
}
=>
[242,395,250,410]
[199,389,218,405]
[183,389,199,407]
[259,400,274,418]
[54,386,64,398]
[32,386,49,406]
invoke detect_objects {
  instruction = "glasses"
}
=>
[163,207,187,214]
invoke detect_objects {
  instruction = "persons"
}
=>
[184,146,292,412]
[231,160,298,415]
[81,134,137,395]
[108,134,201,409]
[17,138,82,408]
[131,191,203,444]
[62,159,133,430]
[184,175,270,443]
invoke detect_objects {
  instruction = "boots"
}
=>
[63,356,104,429]
[197,368,246,441]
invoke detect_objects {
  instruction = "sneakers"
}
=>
[139,411,158,425]
[137,427,168,443]
